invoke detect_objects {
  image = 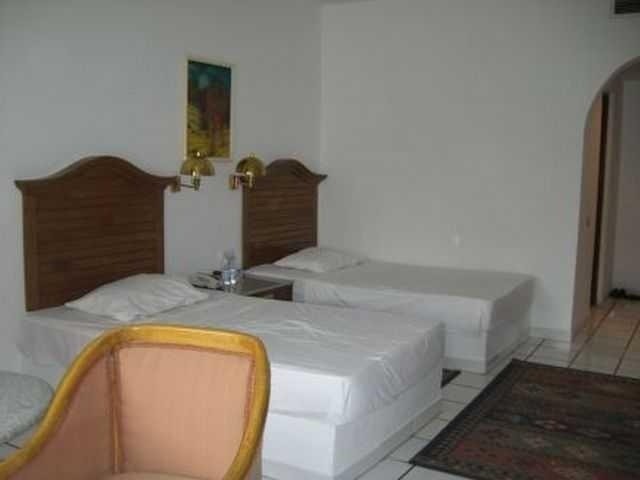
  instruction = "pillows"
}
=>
[273,246,367,274]
[62,272,209,323]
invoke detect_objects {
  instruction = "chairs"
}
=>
[0,324,272,480]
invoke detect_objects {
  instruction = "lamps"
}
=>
[170,150,268,193]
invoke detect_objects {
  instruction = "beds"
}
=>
[243,159,537,373]
[14,155,447,478]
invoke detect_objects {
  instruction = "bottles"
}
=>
[224,249,239,287]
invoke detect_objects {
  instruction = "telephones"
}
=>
[187,268,223,292]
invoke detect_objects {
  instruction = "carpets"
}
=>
[409,357,639,480]
